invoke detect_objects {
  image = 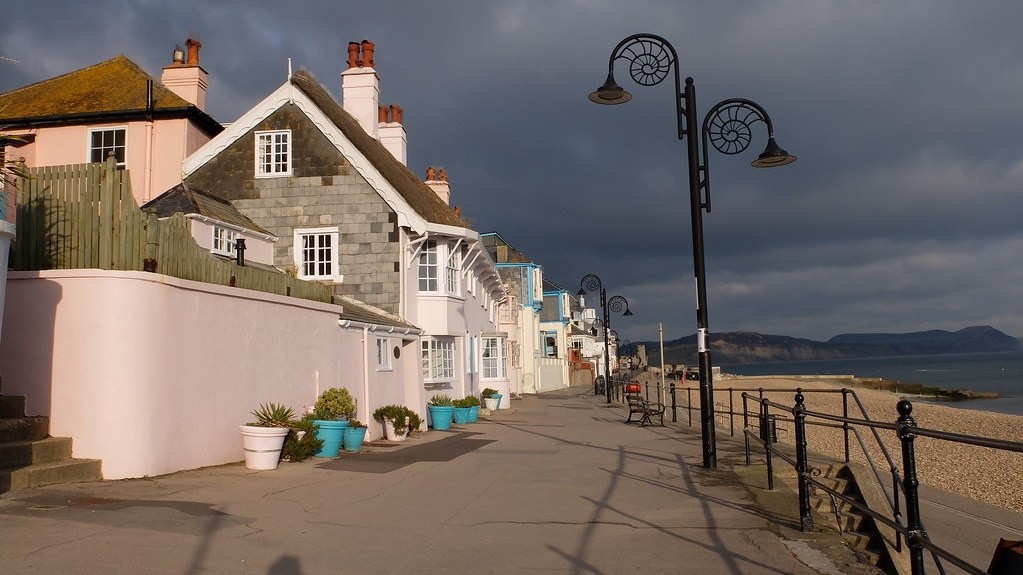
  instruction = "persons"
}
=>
[656,371,660,380]
[673,369,678,382]
[681,368,687,384]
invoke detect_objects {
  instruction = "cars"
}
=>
[668,371,700,381]
[626,381,641,394]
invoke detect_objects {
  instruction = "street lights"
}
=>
[590,319,620,384]
[588,34,799,471]
[575,274,635,404]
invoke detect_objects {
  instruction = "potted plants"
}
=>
[373,405,423,443]
[482,388,504,411]
[341,420,369,453]
[306,386,356,458]
[238,402,296,471]
[465,396,481,423]
[278,417,322,462]
[453,399,471,424]
[427,395,455,431]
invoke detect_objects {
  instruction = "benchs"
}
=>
[624,395,667,428]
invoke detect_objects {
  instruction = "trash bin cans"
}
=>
[595,375,606,395]
